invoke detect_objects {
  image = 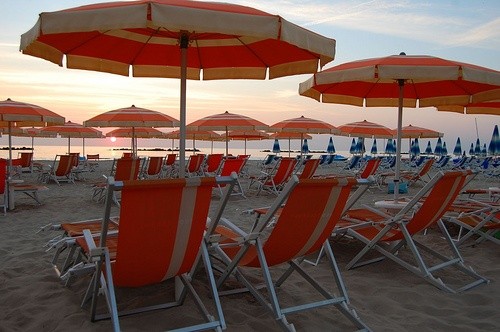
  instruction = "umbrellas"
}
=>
[487,124,500,160]
[273,136,487,171]
[298,52,500,202]
[0,98,445,171]
[19,0,337,177]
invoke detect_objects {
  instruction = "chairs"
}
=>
[0,152,500,332]
